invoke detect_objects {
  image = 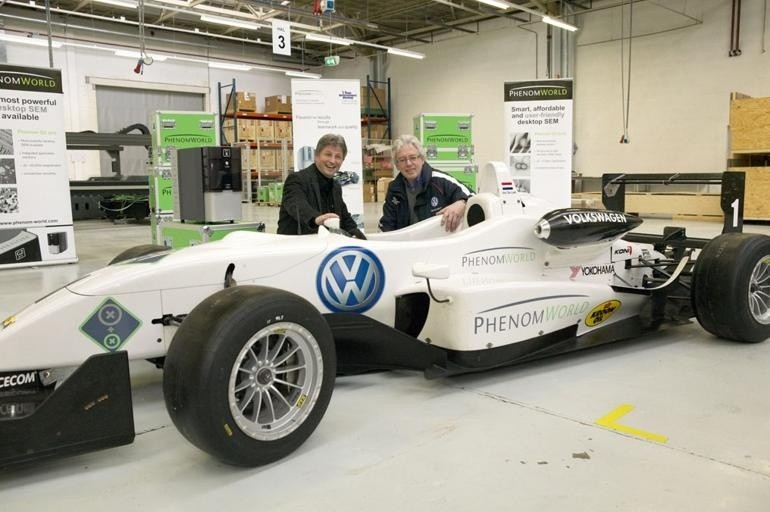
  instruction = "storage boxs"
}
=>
[413,113,478,164]
[147,111,265,250]
[436,164,479,194]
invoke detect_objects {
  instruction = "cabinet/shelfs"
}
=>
[219,75,392,204]
[221,86,394,204]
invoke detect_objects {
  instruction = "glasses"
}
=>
[397,154,417,164]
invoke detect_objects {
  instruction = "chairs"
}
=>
[460,192,501,232]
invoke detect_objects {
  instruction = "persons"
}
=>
[277,132,367,240]
[376,133,477,231]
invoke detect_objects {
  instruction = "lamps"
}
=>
[479,0,511,10]
[0,0,427,79]
[542,17,578,33]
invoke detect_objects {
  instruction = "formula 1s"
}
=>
[2,159,769,470]
